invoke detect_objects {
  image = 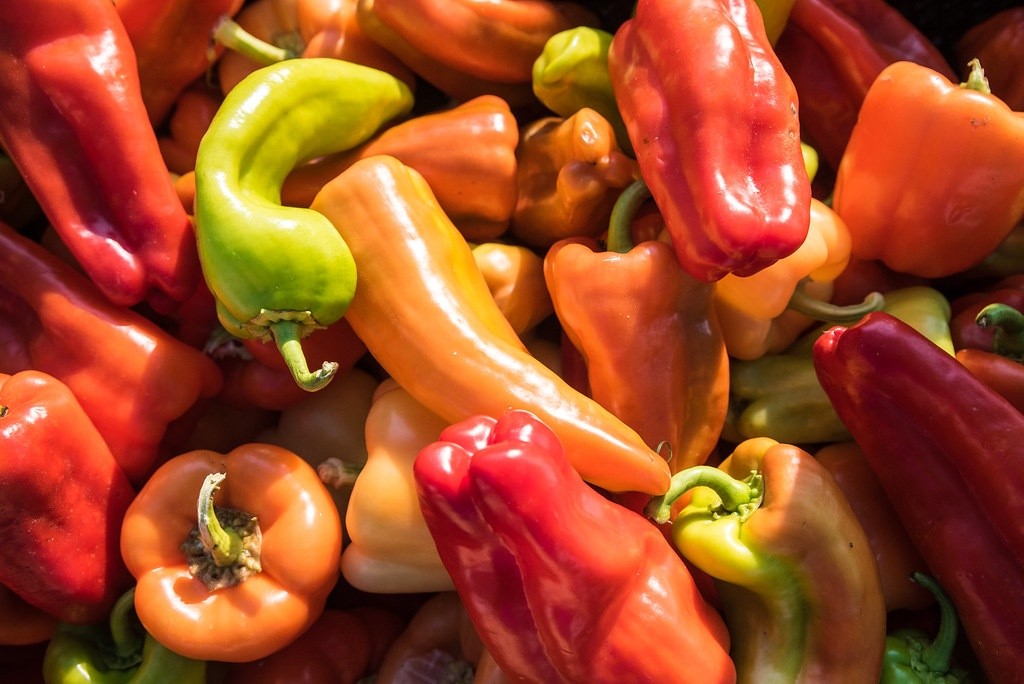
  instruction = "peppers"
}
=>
[0,0,1024,684]
